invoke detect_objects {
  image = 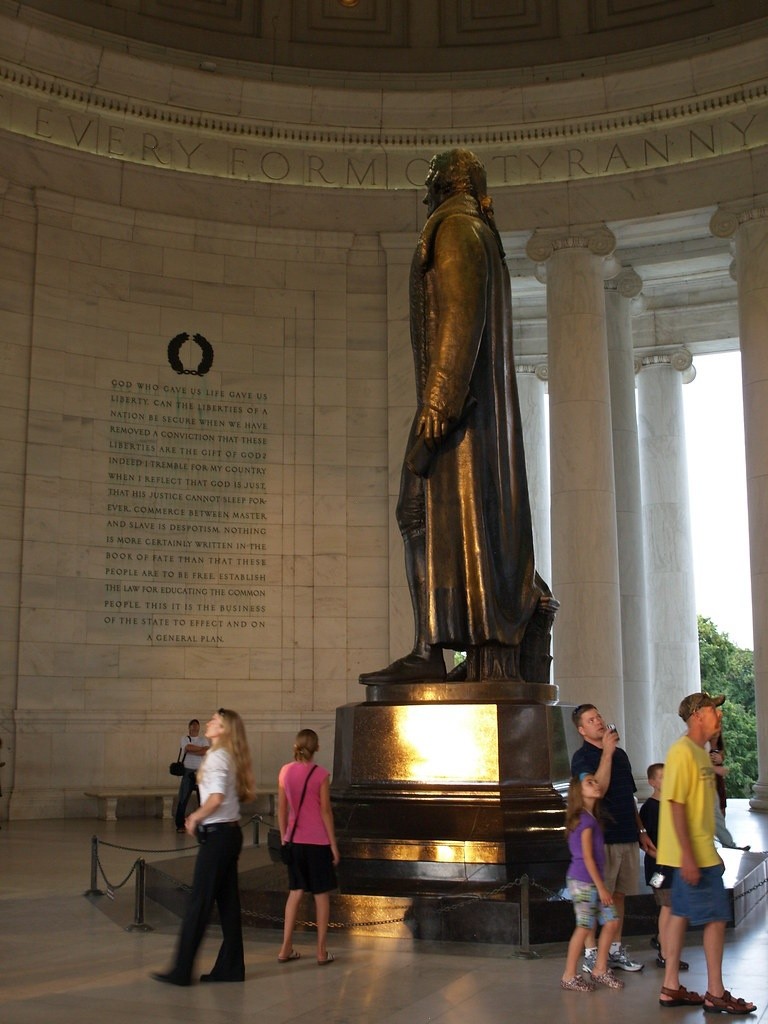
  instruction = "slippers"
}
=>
[318,950,335,966]
[278,950,302,961]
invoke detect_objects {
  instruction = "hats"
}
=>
[679,692,726,720]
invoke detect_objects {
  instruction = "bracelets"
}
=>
[640,829,646,833]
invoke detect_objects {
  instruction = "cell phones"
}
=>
[608,724,620,741]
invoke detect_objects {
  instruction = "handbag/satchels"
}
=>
[170,762,185,776]
[279,841,302,864]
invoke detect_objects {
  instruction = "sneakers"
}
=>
[581,950,598,972]
[607,943,645,970]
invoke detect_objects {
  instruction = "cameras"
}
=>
[649,873,665,888]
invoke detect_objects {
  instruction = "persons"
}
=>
[175,719,210,834]
[640,763,690,970]
[650,693,757,1014]
[571,704,657,976]
[148,708,256,987]
[357,149,561,683]
[561,771,625,993]
[277,728,340,965]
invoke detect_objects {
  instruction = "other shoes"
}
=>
[560,975,596,992]
[655,955,689,969]
[176,827,186,833]
[150,973,192,986]
[651,937,661,949]
[733,846,750,851]
[592,967,625,989]
[200,974,245,982]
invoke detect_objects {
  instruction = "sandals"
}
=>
[659,985,705,1007]
[702,990,757,1014]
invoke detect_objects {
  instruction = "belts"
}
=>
[198,821,236,833]
